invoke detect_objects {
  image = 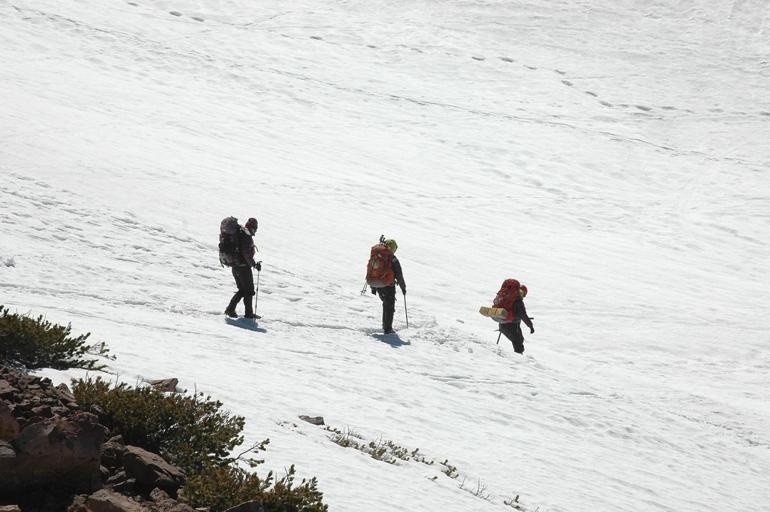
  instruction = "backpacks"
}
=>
[493,278,521,311]
[218,216,244,267]
[366,244,394,285]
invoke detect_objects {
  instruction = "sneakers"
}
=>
[225,309,237,318]
[245,314,261,319]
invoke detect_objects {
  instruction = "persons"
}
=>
[492,278,534,355]
[365,238,406,336]
[225,218,261,319]
